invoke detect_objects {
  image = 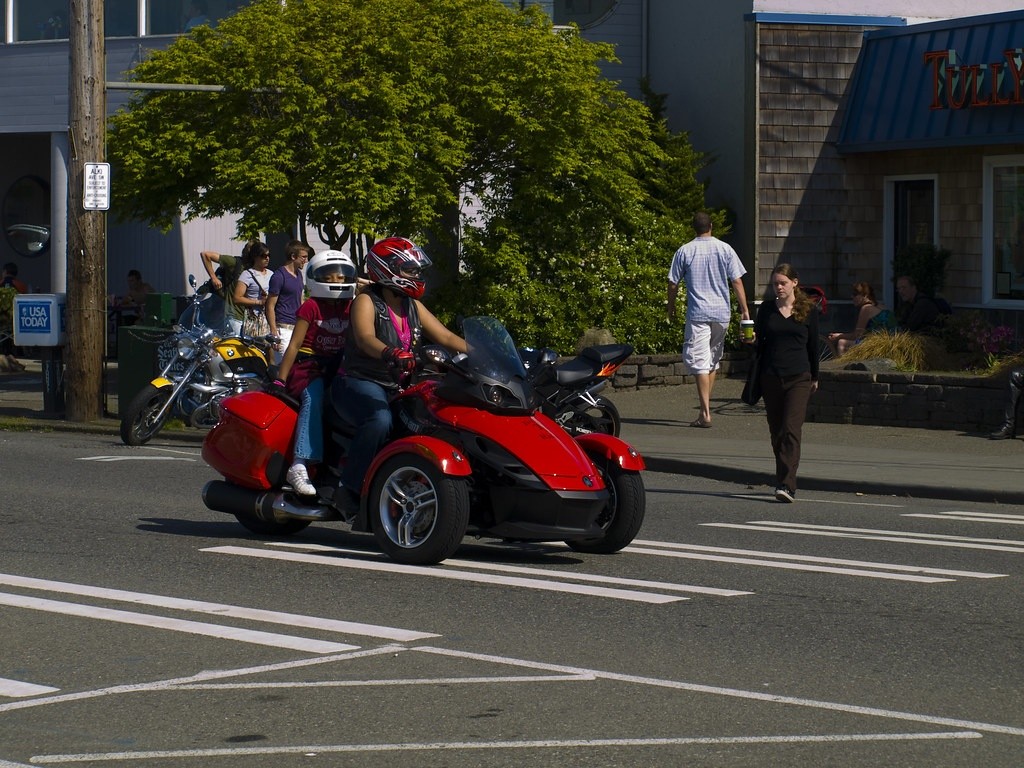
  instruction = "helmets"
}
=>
[305,250,358,299]
[367,237,425,299]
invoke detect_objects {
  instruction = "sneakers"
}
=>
[286,464,316,495]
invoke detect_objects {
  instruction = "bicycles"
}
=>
[819,333,836,363]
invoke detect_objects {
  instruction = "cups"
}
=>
[740,319,754,340]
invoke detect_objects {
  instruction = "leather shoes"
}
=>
[990,425,1015,439]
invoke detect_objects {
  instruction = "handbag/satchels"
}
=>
[195,256,241,293]
[241,292,271,351]
[741,354,762,405]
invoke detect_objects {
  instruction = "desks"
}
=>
[108,306,143,362]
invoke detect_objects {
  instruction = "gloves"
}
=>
[382,346,415,370]
[266,378,285,400]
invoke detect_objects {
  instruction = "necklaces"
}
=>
[385,298,401,315]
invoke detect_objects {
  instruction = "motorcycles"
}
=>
[121,274,281,444]
[518,342,635,438]
[200,314,648,567]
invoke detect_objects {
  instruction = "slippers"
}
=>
[690,419,712,427]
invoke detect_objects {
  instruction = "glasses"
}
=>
[257,253,270,258]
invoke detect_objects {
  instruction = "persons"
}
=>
[108,269,155,325]
[991,369,1024,440]
[266,236,476,498]
[0,262,26,293]
[828,274,958,358]
[198,240,311,360]
[666,210,751,428]
[739,261,819,503]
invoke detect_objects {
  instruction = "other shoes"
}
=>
[775,485,794,502]
